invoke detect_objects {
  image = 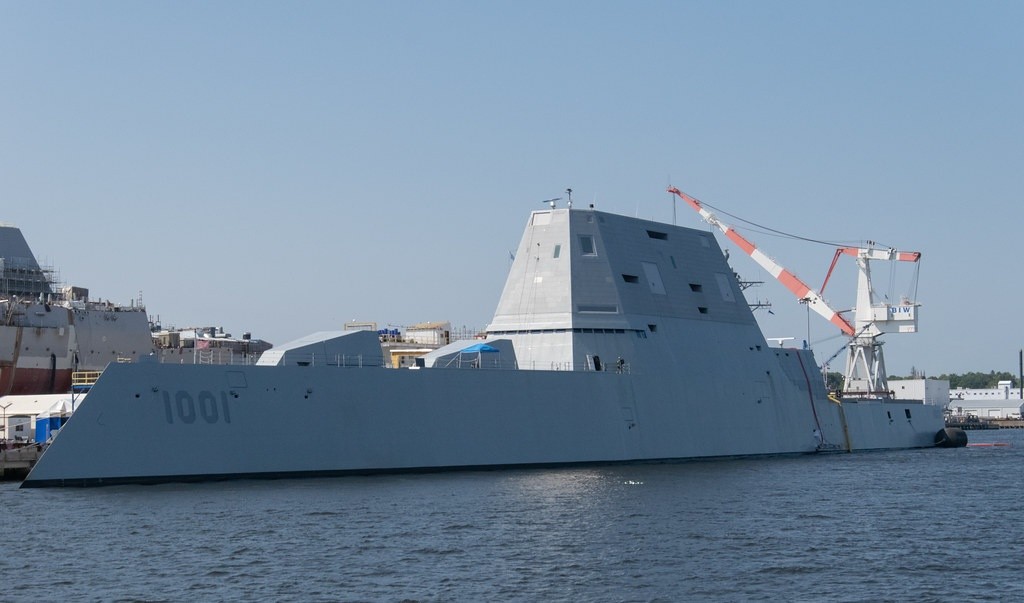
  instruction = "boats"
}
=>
[24,185,969,485]
[0,227,274,479]
[945,412,1000,430]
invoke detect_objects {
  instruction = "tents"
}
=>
[462,342,500,368]
